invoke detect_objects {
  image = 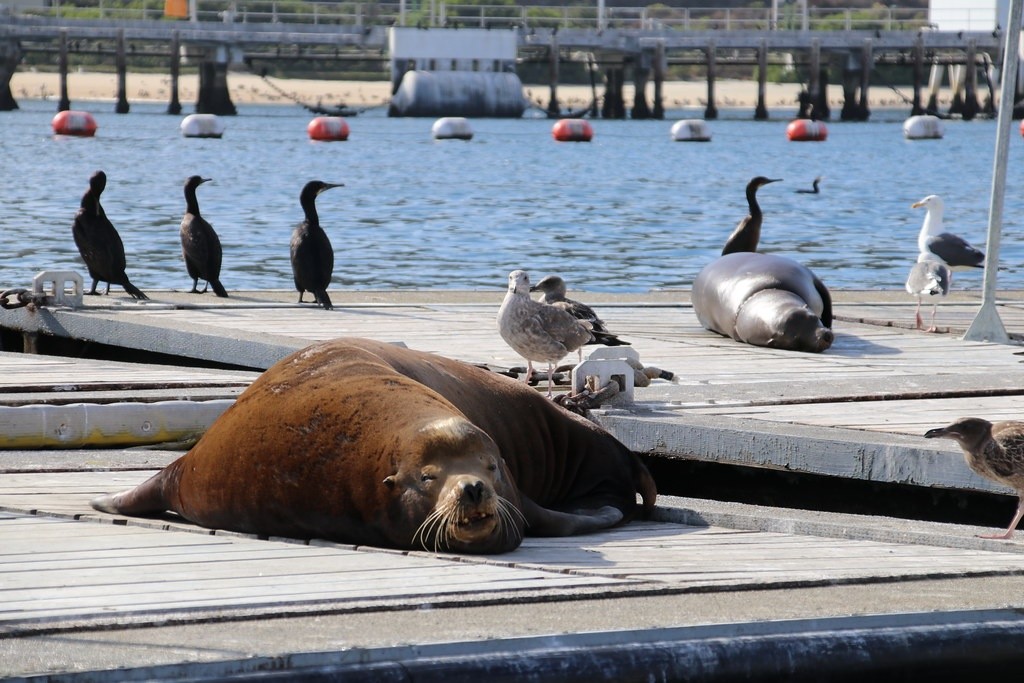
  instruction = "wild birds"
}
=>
[72,171,149,300]
[723,177,783,256]
[924,417,1024,539]
[796,176,823,194]
[289,181,346,311]
[497,269,608,395]
[911,195,1009,277]
[530,276,630,363]
[906,235,954,333]
[180,176,228,297]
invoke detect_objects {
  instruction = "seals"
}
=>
[87,335,657,554]
[691,251,833,353]
